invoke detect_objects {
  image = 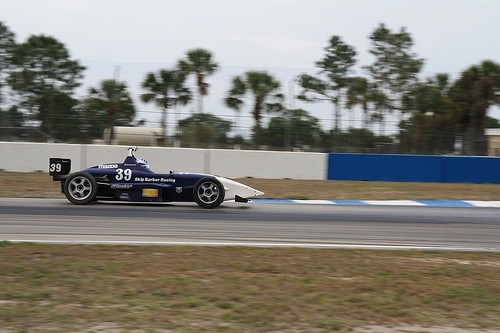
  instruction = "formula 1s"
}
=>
[48,148,266,209]
